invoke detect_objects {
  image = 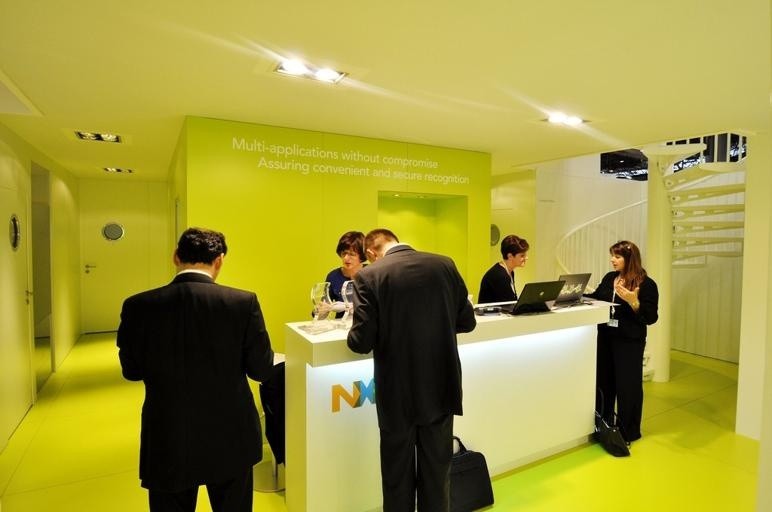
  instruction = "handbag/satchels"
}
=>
[593,411,631,458]
[450,436,494,512]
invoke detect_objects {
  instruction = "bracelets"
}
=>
[630,301,639,309]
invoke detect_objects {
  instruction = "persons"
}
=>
[583,241,658,441]
[478,235,529,302]
[348,230,476,512]
[117,227,274,511]
[314,231,370,320]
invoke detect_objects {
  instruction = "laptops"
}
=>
[487,280,566,315]
[555,273,591,305]
